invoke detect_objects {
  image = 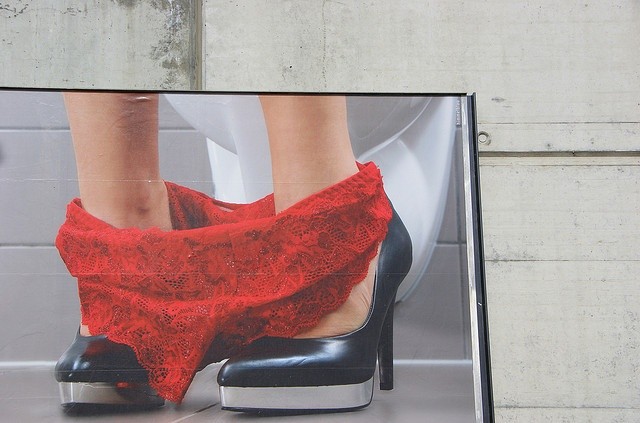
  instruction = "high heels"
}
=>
[216,201,414,413]
[53,319,166,415]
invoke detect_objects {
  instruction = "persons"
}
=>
[55,92,408,410]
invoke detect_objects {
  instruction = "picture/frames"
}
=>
[0,86,496,423]
